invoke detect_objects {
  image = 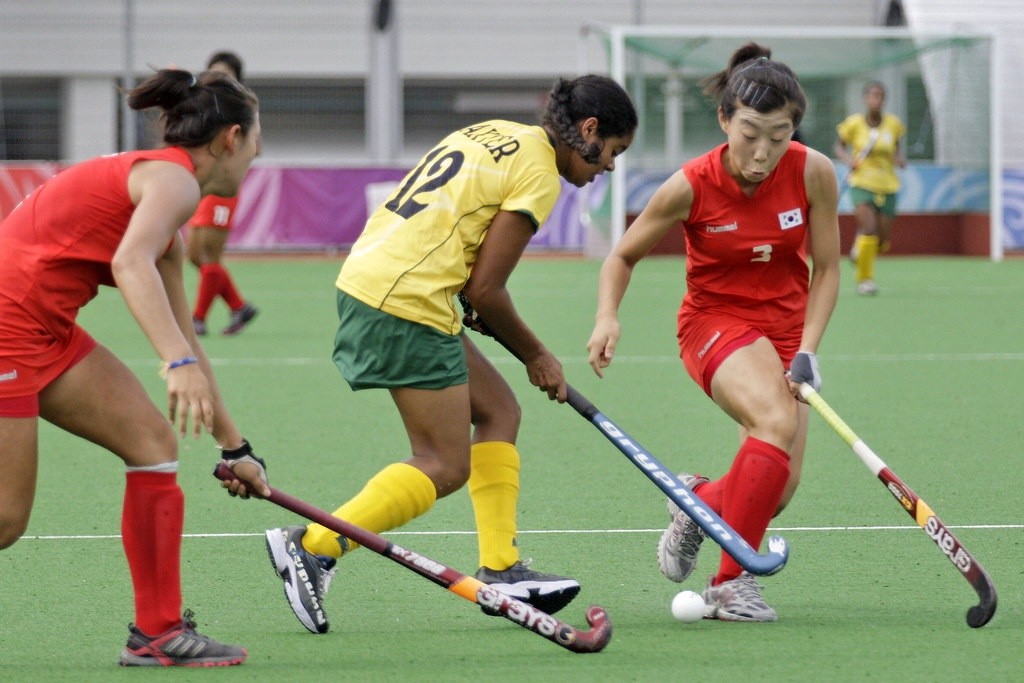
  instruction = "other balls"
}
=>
[671,591,706,625]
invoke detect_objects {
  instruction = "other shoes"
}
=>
[191,319,205,332]
[859,281,880,293]
[223,304,256,334]
[851,243,856,260]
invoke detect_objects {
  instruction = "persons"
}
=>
[0,61,262,667]
[266,73,640,635]
[831,82,908,296]
[587,43,841,621]
[186,53,264,336]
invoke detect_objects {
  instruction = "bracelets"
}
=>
[156,358,197,375]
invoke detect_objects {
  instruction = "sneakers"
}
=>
[657,473,712,584]
[702,570,777,623]
[120,608,247,666]
[264,525,338,634]
[473,555,580,618]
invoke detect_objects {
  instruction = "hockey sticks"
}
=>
[837,128,880,205]
[787,371,998,629]
[211,461,613,654]
[472,307,789,578]
